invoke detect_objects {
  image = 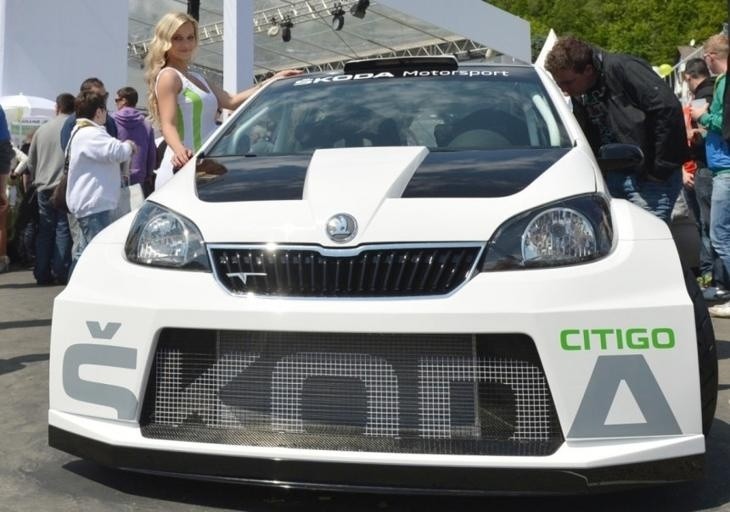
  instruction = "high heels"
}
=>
[0,255,10,272]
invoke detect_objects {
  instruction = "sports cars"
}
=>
[48,55,718,504]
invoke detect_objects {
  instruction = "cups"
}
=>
[691,98,706,128]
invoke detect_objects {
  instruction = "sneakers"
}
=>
[708,300,730,318]
[697,273,729,301]
[37,277,64,285]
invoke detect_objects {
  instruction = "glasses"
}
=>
[115,98,123,101]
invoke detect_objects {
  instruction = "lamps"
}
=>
[279,17,293,41]
[330,0,369,31]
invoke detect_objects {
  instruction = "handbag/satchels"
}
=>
[48,172,72,214]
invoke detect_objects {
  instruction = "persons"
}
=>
[544,36,688,225]
[143,11,305,191]
[0,77,157,286]
[672,34,730,318]
